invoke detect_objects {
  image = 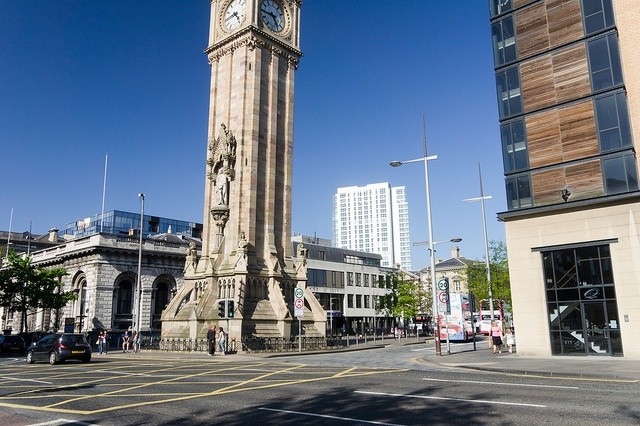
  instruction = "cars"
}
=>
[27,333,91,365]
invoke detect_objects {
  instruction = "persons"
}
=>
[122,330,130,353]
[103,330,109,355]
[217,327,226,356]
[207,324,216,356]
[98,331,103,355]
[504,328,515,353]
[489,322,504,354]
[132,331,138,353]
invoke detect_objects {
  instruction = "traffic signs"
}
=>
[294,287,305,316]
[437,278,450,314]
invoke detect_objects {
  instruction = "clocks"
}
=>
[220,1,247,31]
[261,0,293,36]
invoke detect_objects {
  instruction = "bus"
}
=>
[413,314,430,337]
[479,297,509,335]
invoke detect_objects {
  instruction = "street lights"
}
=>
[430,238,462,355]
[134,193,146,350]
[389,155,442,358]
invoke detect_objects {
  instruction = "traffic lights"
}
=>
[218,301,225,318]
[228,300,234,317]
